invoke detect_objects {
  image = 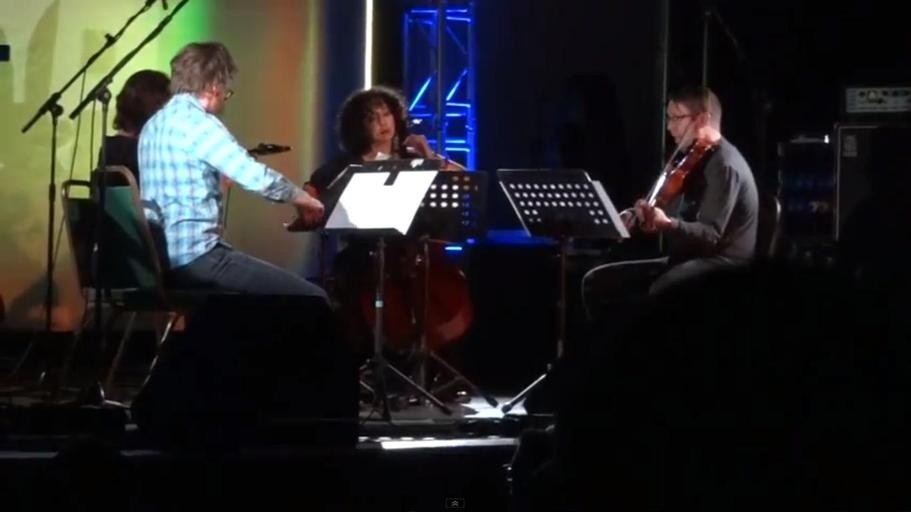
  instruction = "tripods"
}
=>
[393,238,498,412]
[360,235,451,422]
[28,76,189,407]
[502,244,570,414]
[2,93,166,405]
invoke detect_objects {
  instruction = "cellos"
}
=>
[360,120,474,348]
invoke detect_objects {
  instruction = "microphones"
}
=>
[258,142,291,153]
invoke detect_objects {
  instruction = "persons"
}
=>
[80,70,173,410]
[136,42,332,311]
[297,85,475,402]
[581,84,760,323]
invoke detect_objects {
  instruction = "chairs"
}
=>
[754,188,783,259]
[84,161,248,411]
[58,175,183,394]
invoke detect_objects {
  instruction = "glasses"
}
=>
[224,86,234,101]
[664,112,699,123]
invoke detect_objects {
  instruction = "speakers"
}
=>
[835,122,911,249]
[775,136,836,252]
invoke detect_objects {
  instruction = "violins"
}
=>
[642,137,710,231]
